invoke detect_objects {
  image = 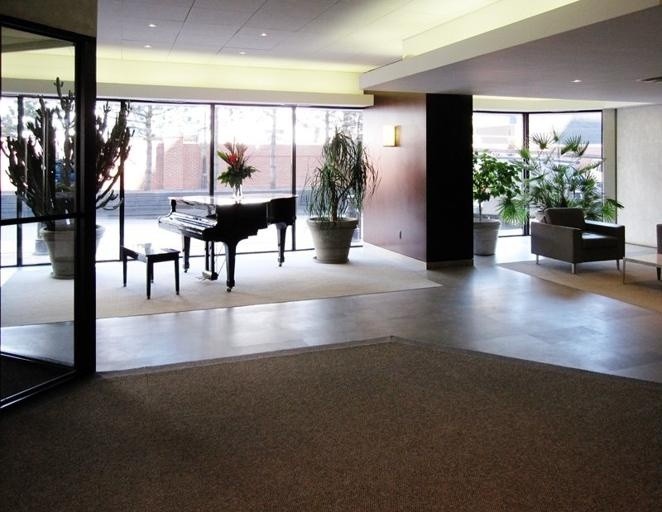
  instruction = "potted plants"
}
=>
[471,146,522,260]
[301,124,383,265]
[0,73,139,284]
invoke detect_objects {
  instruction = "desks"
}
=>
[621,253,660,282]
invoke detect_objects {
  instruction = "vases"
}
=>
[232,181,244,201]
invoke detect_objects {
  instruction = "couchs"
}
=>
[527,207,628,273]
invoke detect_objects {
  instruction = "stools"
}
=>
[122,240,183,300]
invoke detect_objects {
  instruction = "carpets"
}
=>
[2,241,445,329]
[497,254,661,316]
[0,335,662,511]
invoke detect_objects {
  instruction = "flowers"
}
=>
[213,139,255,185]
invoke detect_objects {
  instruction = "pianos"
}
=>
[158,193,299,293]
